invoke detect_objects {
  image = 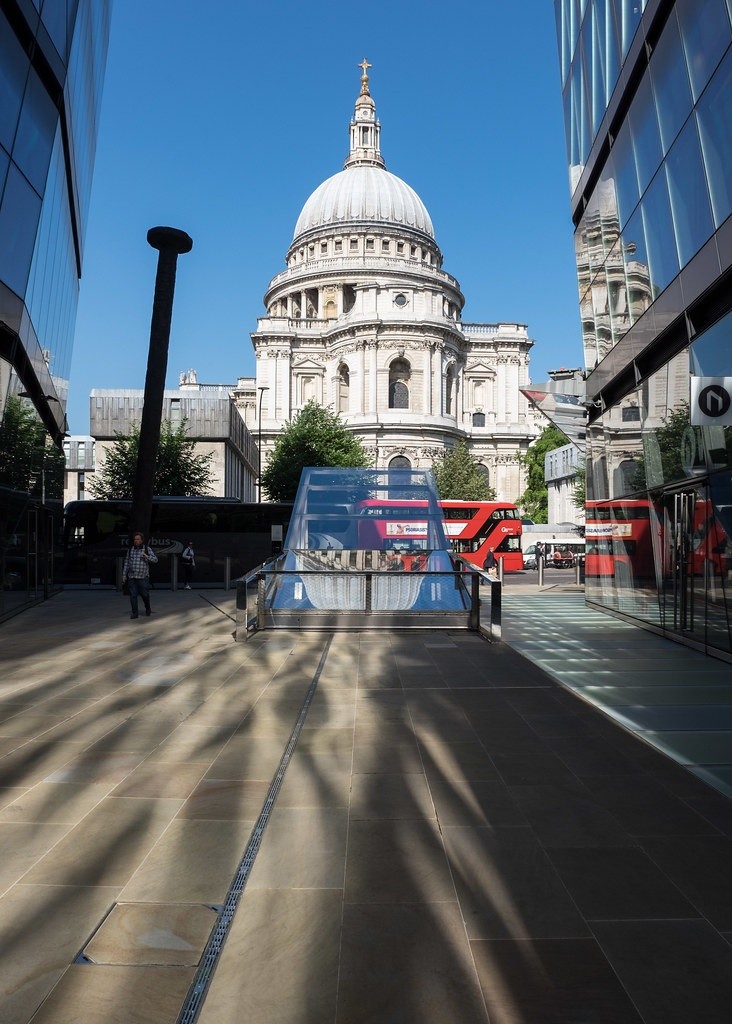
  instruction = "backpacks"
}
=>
[129,545,156,584]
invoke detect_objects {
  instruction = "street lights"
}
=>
[257,387,269,501]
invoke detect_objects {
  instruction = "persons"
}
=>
[479,546,498,586]
[182,541,195,590]
[553,546,574,569]
[122,532,159,619]
[536,542,545,571]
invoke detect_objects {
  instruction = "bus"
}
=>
[60,496,358,582]
[523,539,586,570]
[355,499,523,573]
[585,499,729,579]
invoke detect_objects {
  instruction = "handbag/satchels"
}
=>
[178,547,193,563]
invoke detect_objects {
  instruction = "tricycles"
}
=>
[553,551,576,569]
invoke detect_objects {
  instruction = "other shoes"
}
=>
[146,608,152,616]
[480,581,484,585]
[130,612,138,619]
[186,585,192,590]
